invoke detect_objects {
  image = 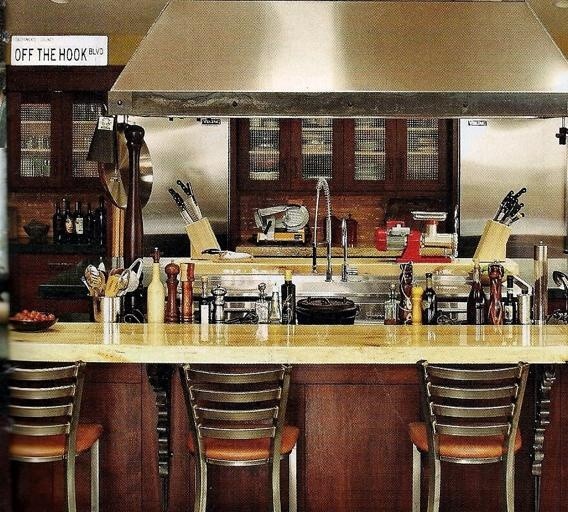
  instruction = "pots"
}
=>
[295,295,361,325]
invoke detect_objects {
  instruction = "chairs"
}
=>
[0,360,104,512]
[407,360,530,512]
[178,364,300,512]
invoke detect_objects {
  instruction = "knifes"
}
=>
[202,247,254,261]
[493,187,531,226]
[167,177,203,225]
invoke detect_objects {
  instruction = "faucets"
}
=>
[339,216,350,283]
[312,177,332,283]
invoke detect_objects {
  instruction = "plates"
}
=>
[247,117,438,185]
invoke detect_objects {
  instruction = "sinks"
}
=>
[300,276,398,298]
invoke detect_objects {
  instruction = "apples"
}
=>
[472,264,509,275]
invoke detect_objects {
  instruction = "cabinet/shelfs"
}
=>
[230,118,343,200]
[0,236,108,322]
[8,65,124,194]
[341,119,452,200]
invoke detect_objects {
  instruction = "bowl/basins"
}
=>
[23,223,50,242]
[7,315,58,332]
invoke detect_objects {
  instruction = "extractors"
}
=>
[108,1,568,123]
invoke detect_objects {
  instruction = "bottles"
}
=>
[198,325,212,346]
[147,322,165,346]
[51,193,108,247]
[197,276,211,325]
[384,262,438,325]
[23,134,53,178]
[465,260,535,325]
[254,269,296,326]
[146,246,165,322]
[211,285,228,323]
